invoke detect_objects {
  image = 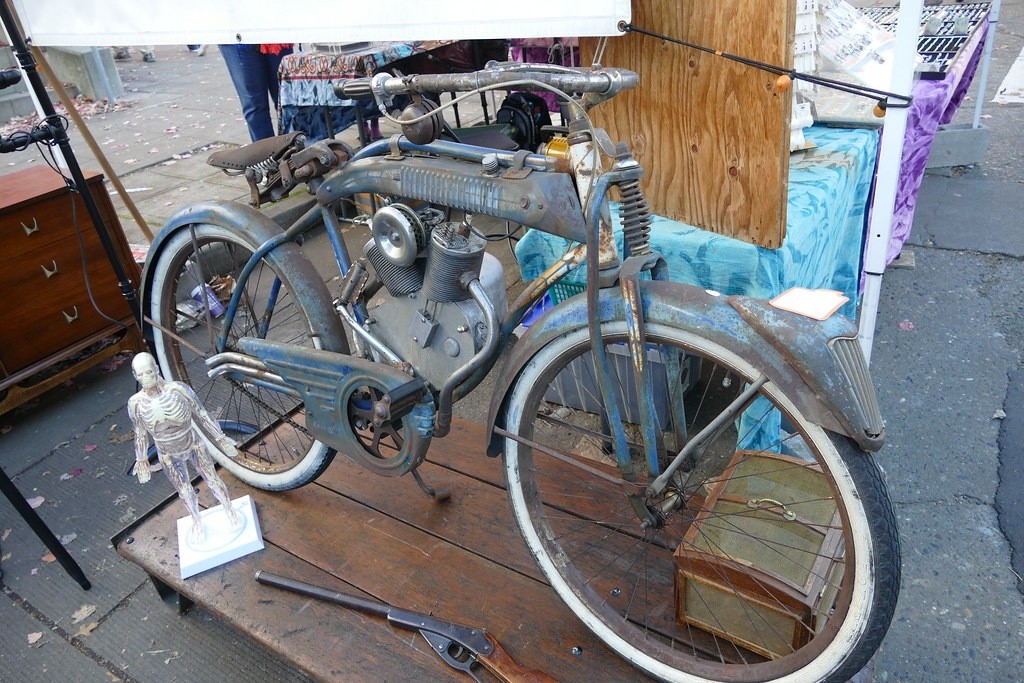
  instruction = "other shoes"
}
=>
[187,44,209,57]
[143,53,156,62]
[364,124,372,138]
[371,128,382,139]
[115,49,131,59]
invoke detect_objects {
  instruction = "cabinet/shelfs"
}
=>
[0,164,145,414]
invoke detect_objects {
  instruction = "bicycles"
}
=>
[137,55,905,683]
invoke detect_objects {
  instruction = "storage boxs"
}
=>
[522,279,703,430]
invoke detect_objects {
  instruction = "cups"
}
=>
[191,283,226,318]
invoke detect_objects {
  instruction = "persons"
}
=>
[218,43,297,185]
[127,353,242,545]
[111,45,157,62]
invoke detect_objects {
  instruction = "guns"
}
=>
[255,569,561,683]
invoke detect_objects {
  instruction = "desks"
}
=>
[510,1,1002,451]
[276,39,484,221]
[109,401,841,683]
